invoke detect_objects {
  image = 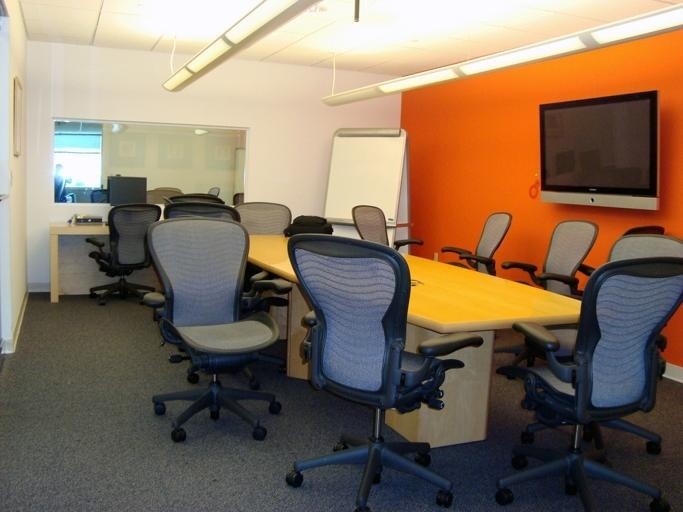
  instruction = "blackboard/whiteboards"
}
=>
[324,128,407,227]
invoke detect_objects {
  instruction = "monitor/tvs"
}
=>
[539,90,661,211]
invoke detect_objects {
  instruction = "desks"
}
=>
[247,233,582,452]
[50,222,110,303]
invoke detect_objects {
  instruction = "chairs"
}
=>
[284,214,334,237]
[439,211,512,278]
[162,202,267,313]
[279,229,460,511]
[232,200,292,282]
[169,194,225,206]
[91,190,108,202]
[351,203,424,254]
[143,216,282,446]
[208,187,220,196]
[605,232,683,267]
[492,220,598,382]
[87,202,158,303]
[621,225,667,357]
[498,255,682,511]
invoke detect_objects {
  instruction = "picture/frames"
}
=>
[13,77,23,156]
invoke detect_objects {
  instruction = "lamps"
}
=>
[322,2,683,106]
[162,0,323,92]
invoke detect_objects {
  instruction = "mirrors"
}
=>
[53,118,250,202]
